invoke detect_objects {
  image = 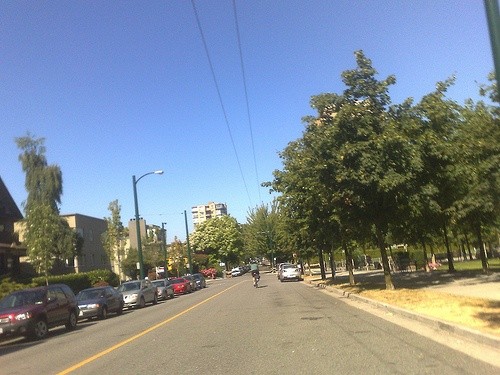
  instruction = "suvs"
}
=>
[1,284,79,340]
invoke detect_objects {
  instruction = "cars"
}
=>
[191,273,206,289]
[279,264,301,282]
[231,265,251,276]
[181,275,198,292]
[75,286,125,319]
[116,279,158,309]
[151,279,174,300]
[278,263,292,279]
[250,264,259,272]
[168,278,191,295]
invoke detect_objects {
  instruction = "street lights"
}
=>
[132,170,166,279]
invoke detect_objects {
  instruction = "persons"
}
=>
[250,260,260,286]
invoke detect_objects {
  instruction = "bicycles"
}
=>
[254,272,258,288]
